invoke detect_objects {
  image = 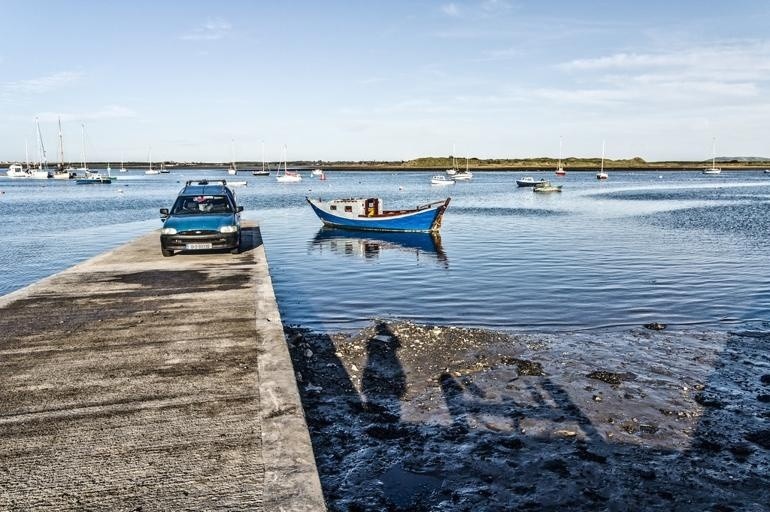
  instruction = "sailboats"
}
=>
[554,135,566,176]
[597,141,609,180]
[430,144,473,186]
[703,136,721,175]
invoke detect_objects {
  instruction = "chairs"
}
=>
[184,202,199,211]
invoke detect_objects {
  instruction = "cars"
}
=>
[159,179,245,257]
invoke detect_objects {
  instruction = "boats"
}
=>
[303,196,452,233]
[516,176,564,193]
[306,224,450,274]
